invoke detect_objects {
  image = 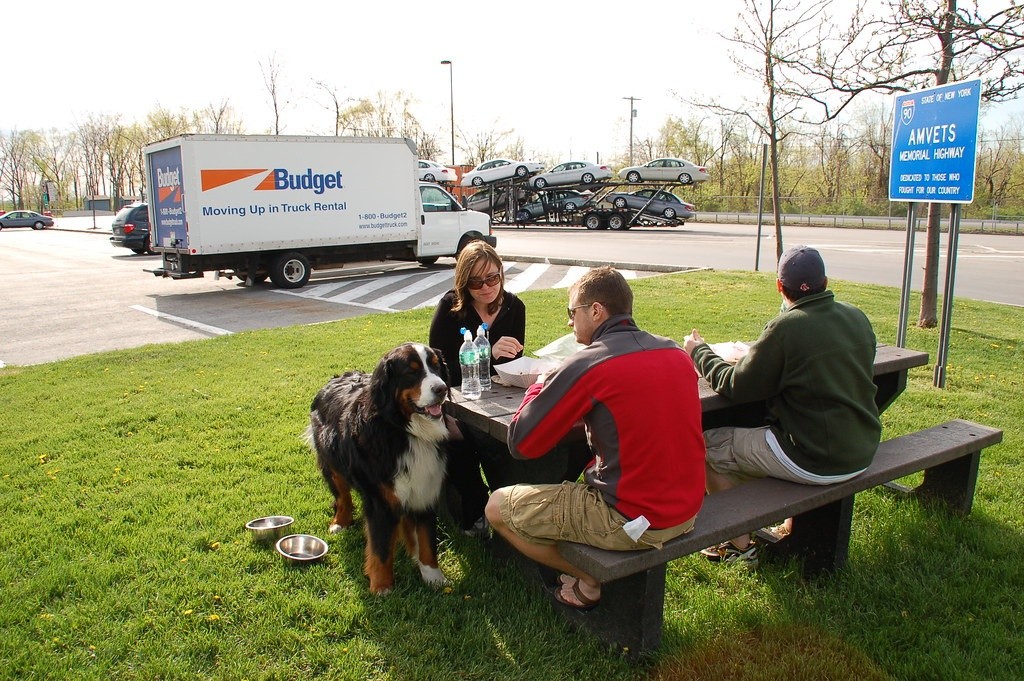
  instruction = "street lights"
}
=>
[441,60,455,165]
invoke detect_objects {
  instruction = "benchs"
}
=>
[555,417,1004,674]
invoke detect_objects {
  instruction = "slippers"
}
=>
[555,578,600,608]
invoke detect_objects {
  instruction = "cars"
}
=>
[460,158,545,187]
[526,161,615,189]
[461,188,536,211]
[418,160,458,183]
[605,188,696,220]
[109,202,163,255]
[0,210,55,231]
[618,158,711,185]
[514,189,595,226]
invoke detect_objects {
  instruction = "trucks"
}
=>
[143,133,498,291]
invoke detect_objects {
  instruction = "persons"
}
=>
[683,245,883,567]
[485,265,707,609]
[428,239,526,541]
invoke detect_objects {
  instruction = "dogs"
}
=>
[295,341,452,598]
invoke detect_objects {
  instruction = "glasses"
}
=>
[467,267,501,289]
[567,301,607,318]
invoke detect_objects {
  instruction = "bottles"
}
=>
[459,328,482,400]
[779,300,788,315]
[474,323,491,391]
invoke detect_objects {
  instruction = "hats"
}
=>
[778,244,823,292]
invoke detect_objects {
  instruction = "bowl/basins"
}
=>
[244,515,294,541]
[276,533,328,565]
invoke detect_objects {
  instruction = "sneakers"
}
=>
[771,524,790,538]
[465,515,489,539]
[700,540,758,565]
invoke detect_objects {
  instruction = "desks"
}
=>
[442,340,930,593]
